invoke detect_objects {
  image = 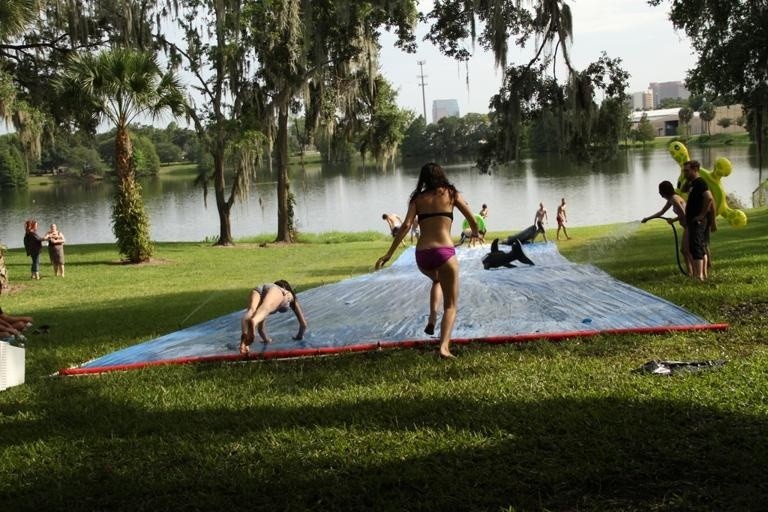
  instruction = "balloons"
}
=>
[669,142,747,228]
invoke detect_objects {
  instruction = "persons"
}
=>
[381,213,406,247]
[23,220,49,280]
[239,278,307,353]
[532,202,549,243]
[556,198,571,241]
[373,161,485,359]
[45,223,65,277]
[410,215,420,245]
[642,160,718,280]
[479,204,489,243]
[0,280,33,338]
